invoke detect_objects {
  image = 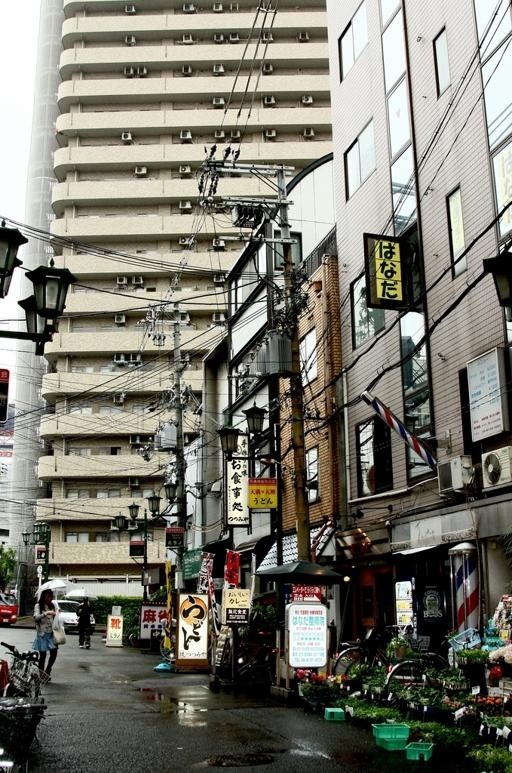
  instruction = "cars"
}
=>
[52,599,96,634]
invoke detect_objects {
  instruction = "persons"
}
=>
[34,587,63,685]
[78,597,94,649]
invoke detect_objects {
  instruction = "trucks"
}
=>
[0,593,17,624]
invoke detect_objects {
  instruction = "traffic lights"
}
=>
[0,369,9,424]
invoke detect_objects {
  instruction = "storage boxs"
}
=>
[324,707,346,721]
[405,742,434,761]
[375,736,408,751]
[372,723,411,738]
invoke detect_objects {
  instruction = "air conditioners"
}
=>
[482,446,512,488]
[182,32,309,43]
[437,455,473,494]
[128,521,138,530]
[125,34,137,46]
[123,4,137,13]
[114,313,227,325]
[135,166,147,176]
[178,200,193,209]
[113,395,124,403]
[122,63,274,76]
[113,353,141,364]
[181,2,223,13]
[178,165,192,174]
[177,237,225,248]
[121,132,132,142]
[213,96,313,107]
[110,521,119,531]
[213,274,225,284]
[180,128,315,139]
[116,273,144,285]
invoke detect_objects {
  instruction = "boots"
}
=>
[39,667,52,684]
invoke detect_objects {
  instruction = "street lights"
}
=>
[115,477,187,602]
[23,521,50,582]
[215,399,285,608]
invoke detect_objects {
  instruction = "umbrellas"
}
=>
[64,585,99,601]
[30,577,84,600]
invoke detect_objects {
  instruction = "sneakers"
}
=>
[79,643,90,648]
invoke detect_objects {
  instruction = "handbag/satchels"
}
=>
[53,626,66,645]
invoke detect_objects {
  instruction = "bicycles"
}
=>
[333,624,447,699]
[0,642,52,699]
